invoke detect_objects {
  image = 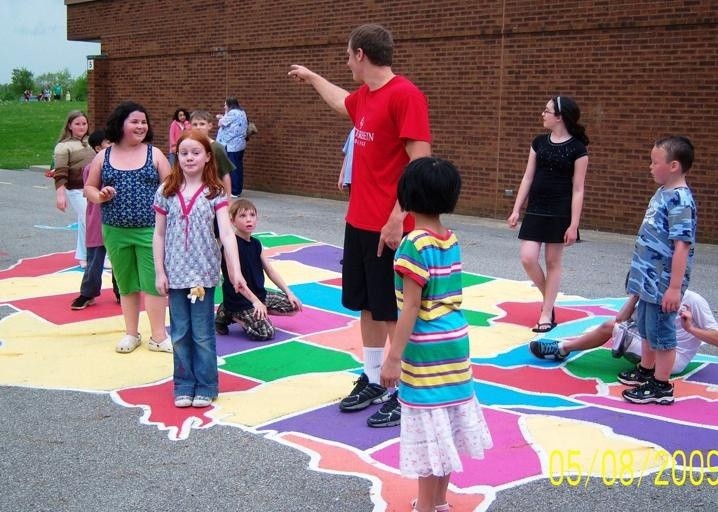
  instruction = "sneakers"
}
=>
[215,303,235,335]
[70,294,97,310]
[338,373,392,412]
[528,341,570,362]
[367,389,402,429]
[617,367,655,386]
[79,257,113,270]
[174,394,215,408]
[622,380,676,405]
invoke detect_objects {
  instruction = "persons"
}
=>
[529,266,717,389]
[288,19,435,428]
[338,124,356,266]
[617,134,701,407]
[378,155,495,510]
[508,93,592,332]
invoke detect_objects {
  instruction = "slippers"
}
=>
[531,301,558,333]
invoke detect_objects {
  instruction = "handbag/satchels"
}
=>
[245,122,258,141]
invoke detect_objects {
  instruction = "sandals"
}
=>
[115,331,142,353]
[147,335,174,353]
